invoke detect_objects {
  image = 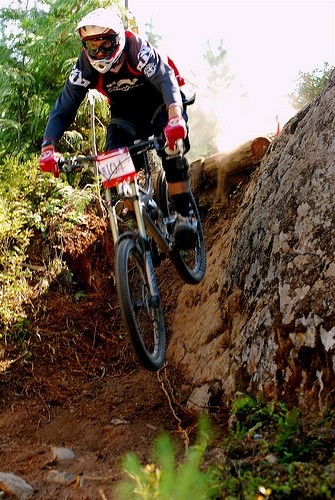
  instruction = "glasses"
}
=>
[85,39,116,56]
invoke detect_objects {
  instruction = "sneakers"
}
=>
[173,217,198,249]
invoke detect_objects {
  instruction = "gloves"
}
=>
[164,116,187,151]
[39,148,60,177]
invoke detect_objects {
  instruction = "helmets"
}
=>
[73,8,126,73]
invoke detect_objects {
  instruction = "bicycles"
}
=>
[51,128,208,373]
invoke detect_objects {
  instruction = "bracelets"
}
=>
[41,139,54,147]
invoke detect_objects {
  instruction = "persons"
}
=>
[40,8,197,249]
